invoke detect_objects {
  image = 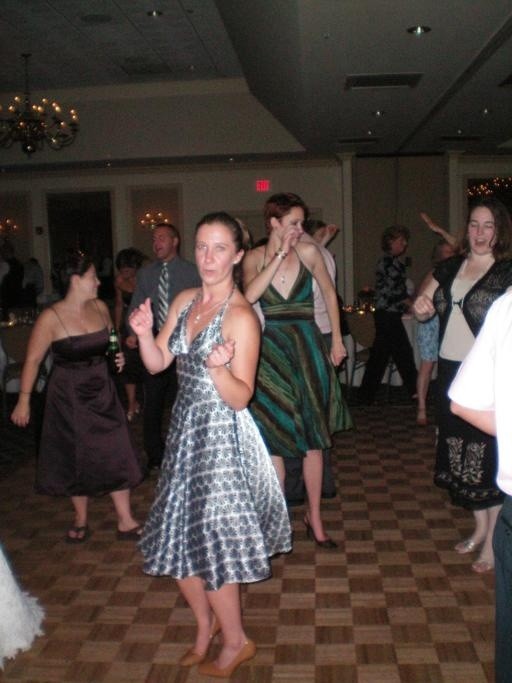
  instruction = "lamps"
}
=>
[0,53,80,153]
[139,209,173,232]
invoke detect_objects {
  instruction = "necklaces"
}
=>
[277,264,288,285]
[193,299,221,325]
[69,307,87,326]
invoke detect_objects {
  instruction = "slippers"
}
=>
[67,523,89,544]
[116,524,155,541]
[458,539,478,556]
[471,554,497,576]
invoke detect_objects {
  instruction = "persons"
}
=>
[354,225,418,410]
[418,207,460,249]
[239,191,348,551]
[305,220,348,337]
[413,239,466,426]
[445,286,512,683]
[113,247,144,339]
[411,198,512,573]
[130,222,203,475]
[11,249,144,543]
[128,209,293,678]
[0,244,23,321]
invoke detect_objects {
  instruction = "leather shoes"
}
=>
[415,412,427,426]
[319,491,336,501]
[287,499,305,506]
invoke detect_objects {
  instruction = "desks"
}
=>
[348,309,421,395]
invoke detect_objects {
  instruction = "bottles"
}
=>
[106,327,121,379]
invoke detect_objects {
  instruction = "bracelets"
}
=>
[277,247,289,257]
[274,252,286,260]
[19,389,32,395]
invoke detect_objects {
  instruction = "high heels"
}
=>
[180,621,221,669]
[197,638,254,679]
[300,519,337,549]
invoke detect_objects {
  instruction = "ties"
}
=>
[156,261,169,333]
